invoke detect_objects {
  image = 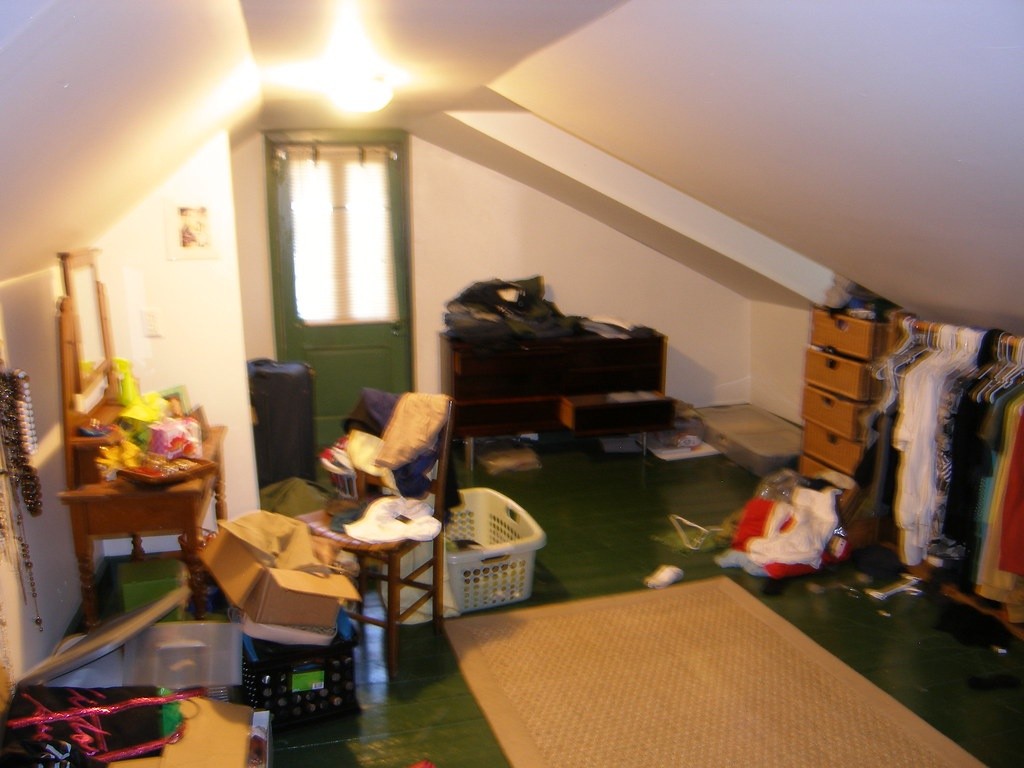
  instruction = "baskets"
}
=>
[237,637,358,731]
[406,487,547,614]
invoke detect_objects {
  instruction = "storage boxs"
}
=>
[693,404,807,481]
[121,513,365,731]
[378,485,548,626]
[799,308,896,553]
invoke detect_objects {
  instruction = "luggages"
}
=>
[249,356,319,487]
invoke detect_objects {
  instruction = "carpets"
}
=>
[443,577,990,768]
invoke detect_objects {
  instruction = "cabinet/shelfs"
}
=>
[435,326,678,438]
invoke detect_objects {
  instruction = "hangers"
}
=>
[873,313,1024,402]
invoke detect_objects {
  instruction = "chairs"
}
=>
[288,386,457,678]
